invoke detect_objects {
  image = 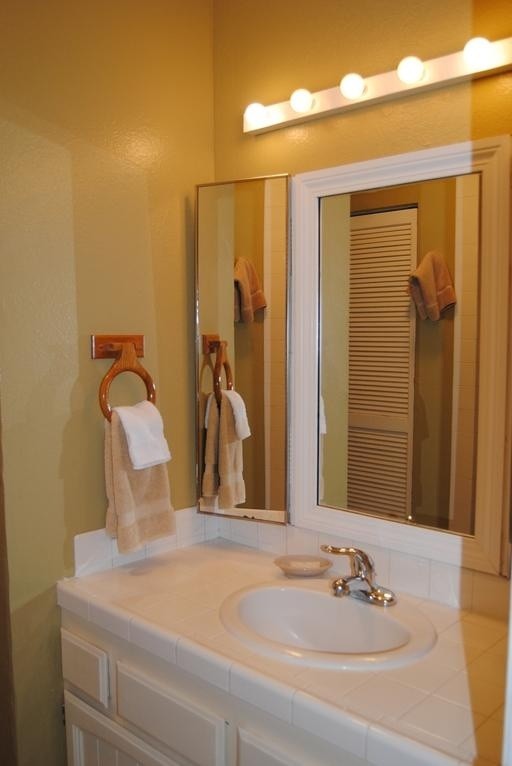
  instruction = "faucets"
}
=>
[321,544,397,608]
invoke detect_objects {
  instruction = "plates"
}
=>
[275,555,332,576]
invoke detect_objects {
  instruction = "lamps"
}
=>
[243,36,512,136]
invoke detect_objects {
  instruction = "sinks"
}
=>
[219,579,436,672]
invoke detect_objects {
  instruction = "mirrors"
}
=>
[195,174,289,525]
[288,135,512,577]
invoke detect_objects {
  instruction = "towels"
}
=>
[103,411,178,556]
[409,251,457,324]
[204,389,251,440]
[234,256,268,325]
[201,393,246,510]
[104,400,172,471]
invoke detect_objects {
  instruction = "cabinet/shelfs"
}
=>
[60,608,373,766]
